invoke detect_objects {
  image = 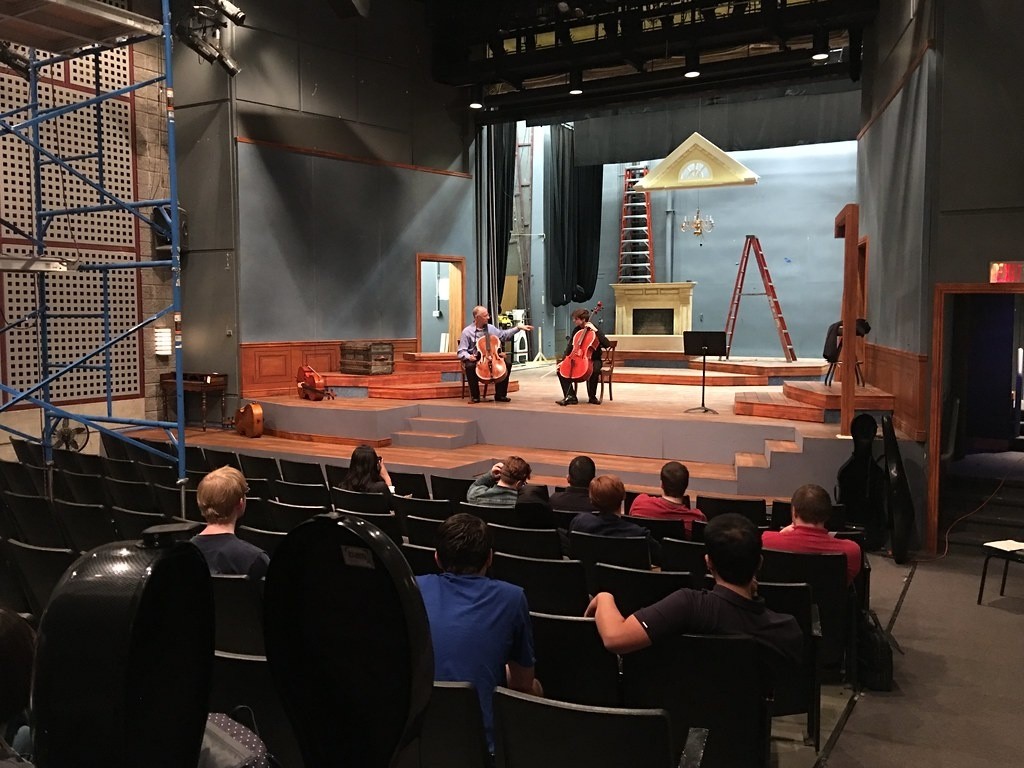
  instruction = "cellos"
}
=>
[296,364,337,401]
[222,400,265,439]
[559,300,603,383]
[475,324,508,385]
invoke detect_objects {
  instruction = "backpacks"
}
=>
[845,608,894,691]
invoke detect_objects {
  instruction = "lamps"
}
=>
[213,46,241,78]
[810,29,830,59]
[210,0,245,27]
[469,84,482,109]
[684,47,701,77]
[154,326,173,356]
[679,186,714,238]
[569,66,583,95]
[178,27,220,65]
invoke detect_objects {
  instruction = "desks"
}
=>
[159,371,228,431]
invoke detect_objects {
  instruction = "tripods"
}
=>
[683,331,726,414]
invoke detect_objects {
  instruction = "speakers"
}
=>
[153,207,189,253]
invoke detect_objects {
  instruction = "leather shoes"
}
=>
[589,397,601,405]
[558,395,578,405]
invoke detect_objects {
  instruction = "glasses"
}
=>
[523,481,528,485]
[376,456,382,462]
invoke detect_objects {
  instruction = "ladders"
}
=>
[718,235,797,363]
[617,167,654,284]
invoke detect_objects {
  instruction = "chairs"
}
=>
[0,429,872,768]
[574,340,618,404]
[976,539,1024,605]
[457,339,497,400]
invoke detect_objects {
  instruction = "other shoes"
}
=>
[495,393,511,402]
[472,396,480,402]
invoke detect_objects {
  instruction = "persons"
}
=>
[551,456,597,511]
[190,467,269,575]
[415,513,544,754]
[466,455,532,505]
[762,484,862,586]
[457,306,533,402]
[0,606,37,718]
[583,514,802,667]
[628,462,708,541]
[340,445,395,514]
[569,474,660,556]
[555,310,609,405]
